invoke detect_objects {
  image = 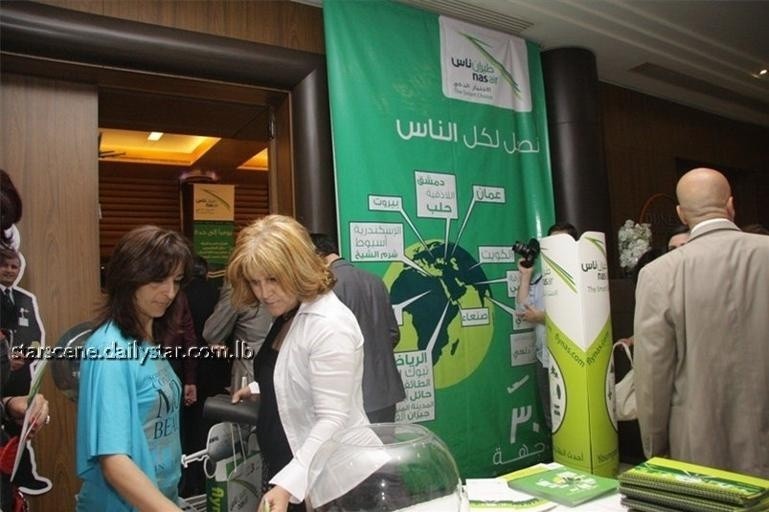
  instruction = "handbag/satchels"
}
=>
[205,394,258,423]
[611,368,639,421]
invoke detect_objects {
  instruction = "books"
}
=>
[618,456,769,511]
[507,466,618,507]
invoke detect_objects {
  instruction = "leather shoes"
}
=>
[16,479,47,490]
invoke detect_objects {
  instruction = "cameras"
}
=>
[511,239,540,269]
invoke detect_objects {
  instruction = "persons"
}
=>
[310,233,407,445]
[2,170,24,510]
[226,214,390,512]
[161,254,274,433]
[512,222,577,430]
[632,167,768,481]
[1,249,54,495]
[75,224,192,512]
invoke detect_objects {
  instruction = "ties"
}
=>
[5,288,13,311]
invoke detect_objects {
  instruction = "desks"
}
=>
[393,462,628,512]
